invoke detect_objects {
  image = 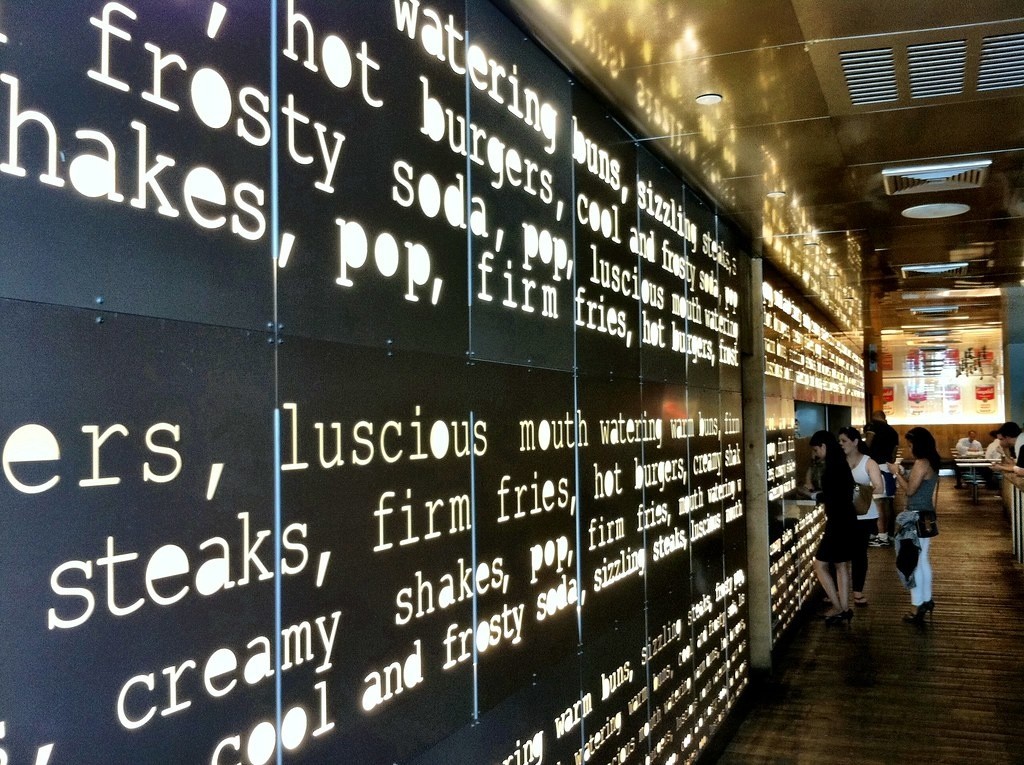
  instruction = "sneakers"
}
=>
[867,537,890,547]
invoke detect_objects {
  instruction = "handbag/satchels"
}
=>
[852,484,873,514]
[913,510,939,538]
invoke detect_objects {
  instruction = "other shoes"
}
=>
[954,486,962,489]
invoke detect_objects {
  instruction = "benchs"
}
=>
[958,469,1003,502]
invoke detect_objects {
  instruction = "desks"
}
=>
[950,447,1003,481]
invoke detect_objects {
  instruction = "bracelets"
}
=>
[893,471,899,478]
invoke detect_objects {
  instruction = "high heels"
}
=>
[903,599,934,623]
[823,609,853,624]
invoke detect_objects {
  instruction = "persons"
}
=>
[953,430,983,489]
[990,422,1024,478]
[983,430,1005,490]
[794,419,802,438]
[838,427,883,606]
[858,410,899,547]
[888,427,941,624]
[797,430,857,625]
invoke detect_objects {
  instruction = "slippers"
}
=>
[855,597,867,606]
